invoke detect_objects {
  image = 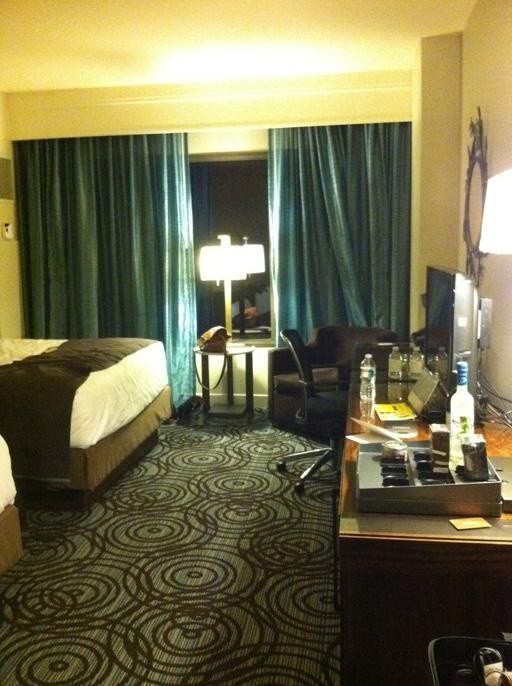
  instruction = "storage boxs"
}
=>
[350,440,503,519]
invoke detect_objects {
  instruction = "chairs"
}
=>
[274,324,351,495]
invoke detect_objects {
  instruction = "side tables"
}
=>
[193,340,258,427]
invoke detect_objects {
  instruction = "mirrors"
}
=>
[464,101,492,290]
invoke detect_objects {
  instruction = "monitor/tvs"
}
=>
[426,264,481,404]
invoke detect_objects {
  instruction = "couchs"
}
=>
[265,322,399,442]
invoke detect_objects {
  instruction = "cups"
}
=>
[380,440,409,461]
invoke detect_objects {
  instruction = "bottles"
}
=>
[463,441,490,481]
[449,360,476,464]
[386,381,407,404]
[359,353,376,400]
[433,346,448,378]
[409,346,425,380]
[360,400,376,434]
[387,345,403,380]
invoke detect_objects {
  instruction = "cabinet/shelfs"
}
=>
[332,366,510,686]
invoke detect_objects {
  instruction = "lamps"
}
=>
[475,168,512,259]
[197,233,250,348]
[238,234,266,342]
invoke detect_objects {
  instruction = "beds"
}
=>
[1,336,175,512]
[2,428,27,579]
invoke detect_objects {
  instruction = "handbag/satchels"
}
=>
[197,325,229,353]
[178,396,209,426]
